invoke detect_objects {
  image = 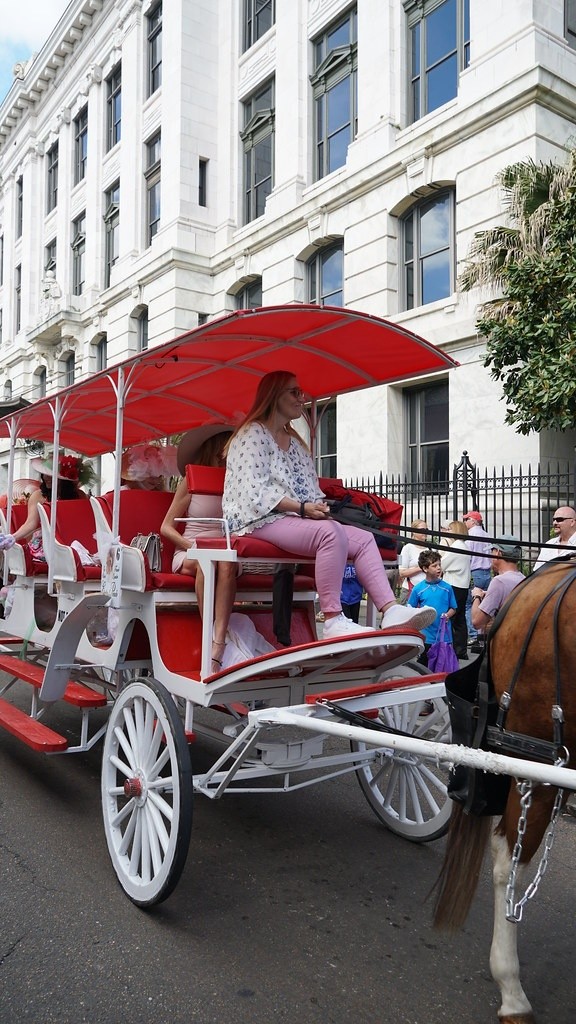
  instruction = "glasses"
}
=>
[553,517,573,522]
[466,518,472,521]
[441,526,445,529]
[422,528,429,530]
[284,388,305,398]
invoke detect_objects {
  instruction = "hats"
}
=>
[461,510,482,523]
[178,423,238,478]
[32,455,99,488]
[483,535,521,556]
[12,479,47,503]
[121,446,181,481]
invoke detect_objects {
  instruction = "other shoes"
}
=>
[419,702,434,715]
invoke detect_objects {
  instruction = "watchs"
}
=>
[471,595,482,605]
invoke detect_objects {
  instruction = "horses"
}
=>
[441,552,576,1024]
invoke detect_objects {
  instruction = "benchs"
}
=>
[0,465,405,611]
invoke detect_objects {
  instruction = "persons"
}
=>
[120,443,182,492]
[406,550,458,716]
[461,512,492,644]
[160,423,243,672]
[469,534,528,630]
[437,519,472,660]
[220,371,438,638]
[0,449,104,594]
[399,519,436,607]
[339,563,363,624]
[533,506,576,572]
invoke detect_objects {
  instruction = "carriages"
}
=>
[0,303,575,1021]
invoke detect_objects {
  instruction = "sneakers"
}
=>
[382,604,436,631]
[323,612,375,639]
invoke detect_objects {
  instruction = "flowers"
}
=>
[127,445,177,480]
[13,491,31,505]
[58,454,94,483]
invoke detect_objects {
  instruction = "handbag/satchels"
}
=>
[326,498,380,530]
[130,533,164,572]
[426,616,459,673]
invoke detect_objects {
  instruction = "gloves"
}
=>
[0,532,15,549]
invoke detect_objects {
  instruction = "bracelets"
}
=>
[300,502,305,519]
